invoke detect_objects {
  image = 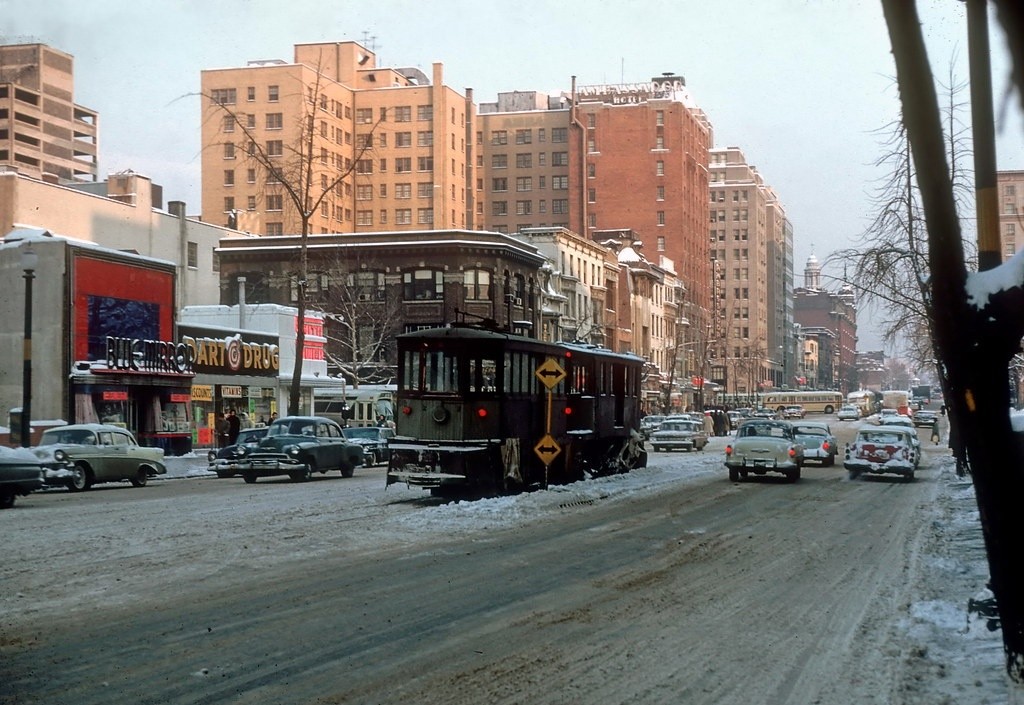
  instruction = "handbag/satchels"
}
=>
[933,434,938,443]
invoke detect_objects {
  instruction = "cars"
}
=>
[230,416,364,484]
[207,425,289,479]
[844,425,921,482]
[723,419,808,483]
[338,426,396,469]
[875,383,943,429]
[837,404,863,421]
[790,421,838,467]
[0,445,47,510]
[640,406,780,453]
[18,423,167,492]
[783,404,808,419]
[649,420,709,451]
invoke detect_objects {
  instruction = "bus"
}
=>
[761,390,843,415]
[847,394,876,417]
[716,392,763,412]
[384,306,653,497]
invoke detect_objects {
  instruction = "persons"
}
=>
[268,412,277,426]
[702,408,732,436]
[377,415,388,427]
[216,413,230,449]
[226,409,256,447]
[940,405,946,417]
[931,418,940,445]
